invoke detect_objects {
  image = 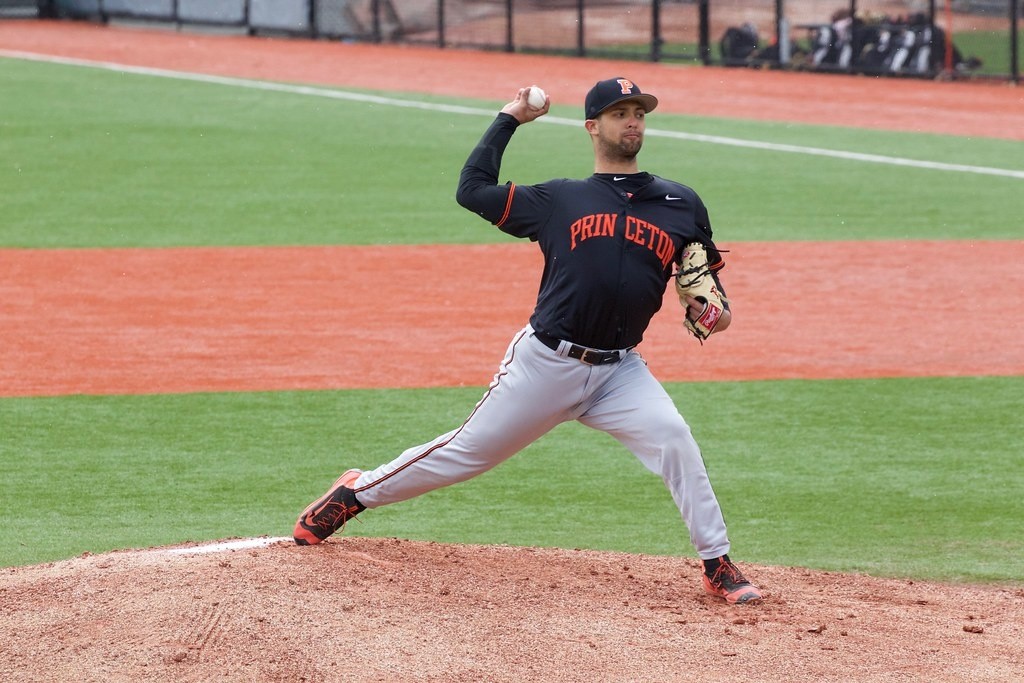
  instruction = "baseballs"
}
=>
[525,85,546,110]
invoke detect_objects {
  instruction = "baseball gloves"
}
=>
[668,242,730,345]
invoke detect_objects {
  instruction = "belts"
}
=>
[533,328,639,365]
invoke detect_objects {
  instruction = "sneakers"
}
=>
[293,468,368,547]
[701,554,762,605]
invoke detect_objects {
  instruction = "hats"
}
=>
[584,76,659,119]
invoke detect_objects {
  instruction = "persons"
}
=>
[290,74,766,608]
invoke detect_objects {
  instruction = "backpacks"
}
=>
[720,11,982,75]
